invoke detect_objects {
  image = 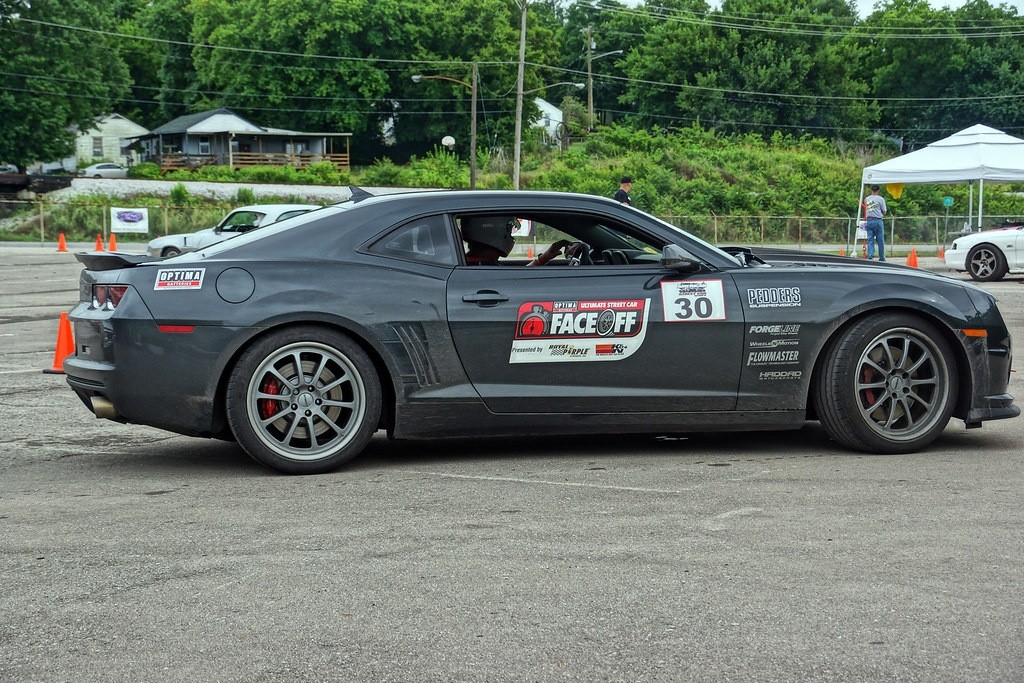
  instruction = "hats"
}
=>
[871,184,879,190]
[621,176,635,183]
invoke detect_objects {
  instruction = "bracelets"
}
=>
[536,253,549,264]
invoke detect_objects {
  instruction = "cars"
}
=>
[77,162,130,179]
[0,164,17,174]
[944,223,1024,282]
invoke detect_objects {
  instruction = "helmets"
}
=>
[461,207,521,257]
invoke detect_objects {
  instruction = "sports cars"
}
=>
[147,203,322,257]
[61,176,1022,474]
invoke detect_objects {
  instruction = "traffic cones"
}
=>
[55,233,68,252]
[108,233,117,252]
[840,248,844,256]
[906,247,918,267]
[938,247,944,259]
[91,233,105,251]
[527,247,534,258]
[42,312,75,376]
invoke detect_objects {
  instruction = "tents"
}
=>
[852,123,1024,257]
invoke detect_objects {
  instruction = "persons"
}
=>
[615,176,633,205]
[863,185,888,262]
[460,216,574,267]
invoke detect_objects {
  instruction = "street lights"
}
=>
[586,50,624,128]
[513,82,585,190]
[412,75,476,190]
